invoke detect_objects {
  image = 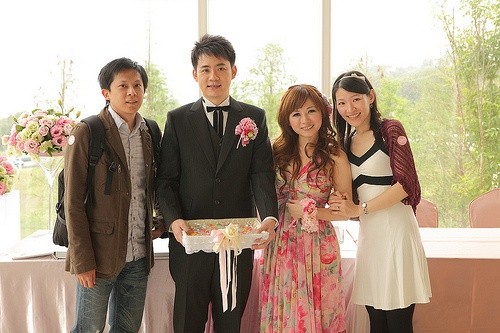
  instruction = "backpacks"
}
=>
[53,116,159,248]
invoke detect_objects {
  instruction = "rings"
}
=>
[337,206,340,210]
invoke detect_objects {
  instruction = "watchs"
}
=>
[362,202,368,214]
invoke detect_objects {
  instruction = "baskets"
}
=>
[181,218,269,254]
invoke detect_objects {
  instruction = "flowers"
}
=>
[0,155,15,195]
[2,102,88,157]
[207,222,241,253]
[301,198,319,236]
[233,117,258,149]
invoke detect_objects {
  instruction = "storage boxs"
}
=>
[181,218,269,254]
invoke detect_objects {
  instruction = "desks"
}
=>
[0,228,500,333]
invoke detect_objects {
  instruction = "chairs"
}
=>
[468,188,500,227]
[414,194,438,228]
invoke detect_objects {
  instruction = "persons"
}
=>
[257,84,353,333]
[63,57,166,333]
[155,34,280,333]
[329,71,432,333]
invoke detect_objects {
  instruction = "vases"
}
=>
[27,145,67,232]
[0,188,22,254]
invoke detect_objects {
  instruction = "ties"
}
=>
[202,99,232,137]
